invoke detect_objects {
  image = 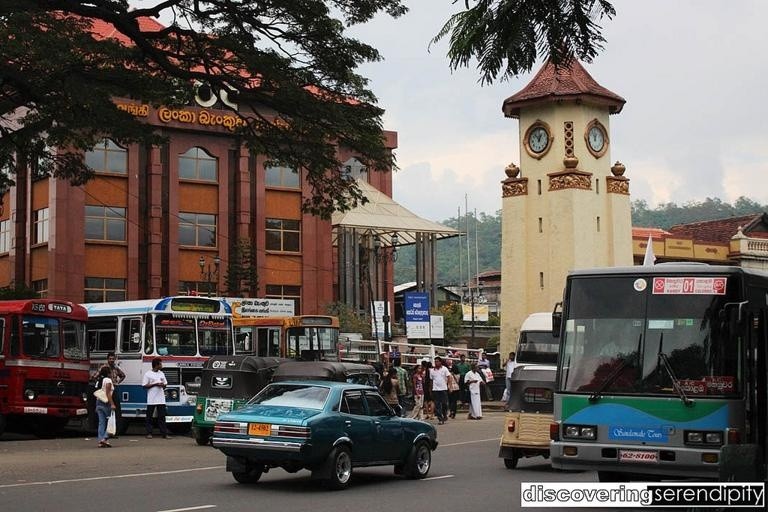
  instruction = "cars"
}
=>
[208,377,440,489]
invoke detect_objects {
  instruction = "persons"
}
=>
[376,350,494,424]
[502,351,515,408]
[142,357,173,438]
[92,352,126,439]
[93,366,117,447]
[600,324,643,367]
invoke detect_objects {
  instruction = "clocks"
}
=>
[584,118,609,158]
[523,119,554,160]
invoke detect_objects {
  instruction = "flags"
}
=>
[643,237,658,267]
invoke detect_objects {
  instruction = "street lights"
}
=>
[198,254,220,296]
[459,280,486,347]
[370,230,402,352]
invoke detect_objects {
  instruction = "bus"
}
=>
[513,311,679,376]
[77,294,238,436]
[0,294,97,434]
[545,257,766,483]
[229,313,342,368]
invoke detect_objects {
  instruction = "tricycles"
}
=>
[189,350,287,449]
[495,363,570,472]
[268,359,385,391]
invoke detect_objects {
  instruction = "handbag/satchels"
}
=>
[481,367,494,383]
[93,388,109,403]
